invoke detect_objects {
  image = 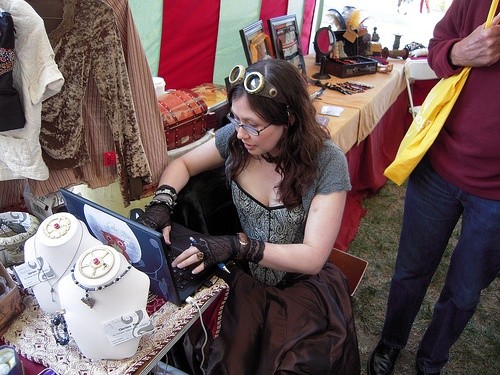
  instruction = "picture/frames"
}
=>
[267,14,306,78]
[240,19,270,67]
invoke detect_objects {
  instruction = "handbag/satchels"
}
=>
[384,69,470,186]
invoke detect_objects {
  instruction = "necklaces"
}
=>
[71,260,133,309]
[34,219,83,302]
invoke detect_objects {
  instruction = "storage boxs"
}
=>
[151,76,232,152]
[329,29,378,77]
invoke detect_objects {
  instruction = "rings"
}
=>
[197,252,204,259]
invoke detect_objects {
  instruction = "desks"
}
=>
[302,49,407,249]
[0,266,225,375]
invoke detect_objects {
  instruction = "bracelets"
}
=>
[154,185,179,202]
[52,313,70,346]
[237,232,248,253]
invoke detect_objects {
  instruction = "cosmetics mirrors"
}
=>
[312,27,336,79]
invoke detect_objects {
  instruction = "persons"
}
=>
[366,0,500,375]
[135,58,361,375]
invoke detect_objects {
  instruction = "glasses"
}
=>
[226,110,273,136]
[229,63,283,101]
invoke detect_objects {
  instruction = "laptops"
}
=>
[59,187,232,306]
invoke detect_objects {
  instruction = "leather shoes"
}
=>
[415,358,440,375]
[367,340,399,375]
